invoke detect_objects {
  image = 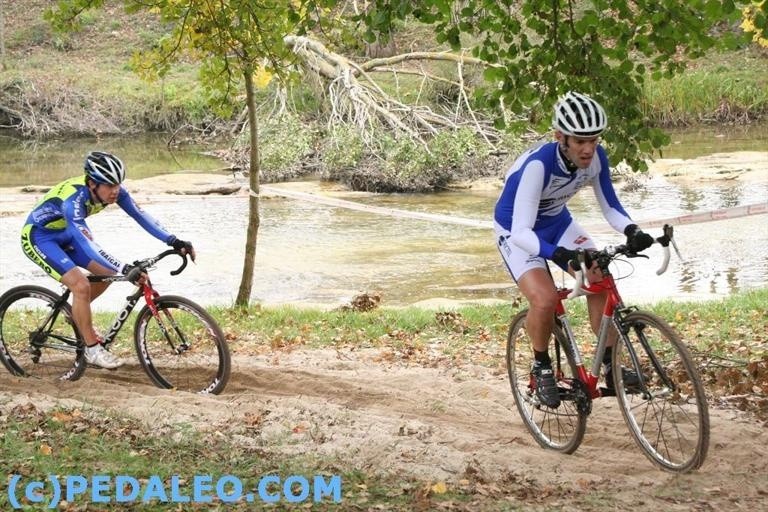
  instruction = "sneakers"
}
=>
[531,362,561,408]
[604,362,639,388]
[83,343,125,369]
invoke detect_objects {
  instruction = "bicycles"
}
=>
[502,220,712,484]
[0,248,231,396]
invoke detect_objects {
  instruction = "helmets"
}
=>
[84,151,125,185]
[553,92,608,137]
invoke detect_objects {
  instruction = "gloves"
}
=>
[624,224,654,253]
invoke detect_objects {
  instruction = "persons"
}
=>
[492,89,655,410]
[20,151,196,370]
